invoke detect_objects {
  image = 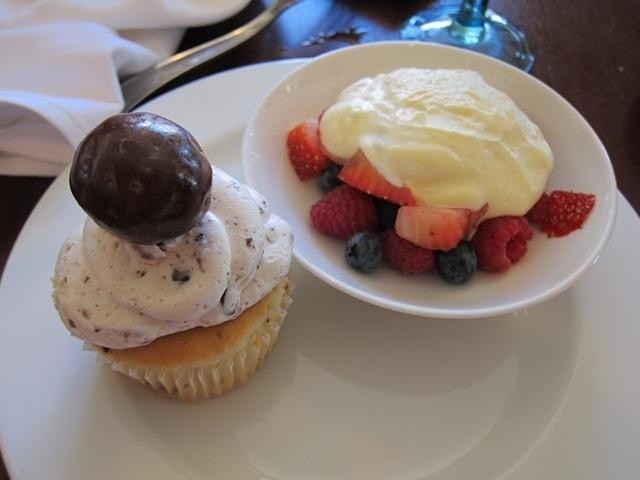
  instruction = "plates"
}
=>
[0,57,640,479]
[242,41,617,320]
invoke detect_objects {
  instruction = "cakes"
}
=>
[51,112,295,404]
